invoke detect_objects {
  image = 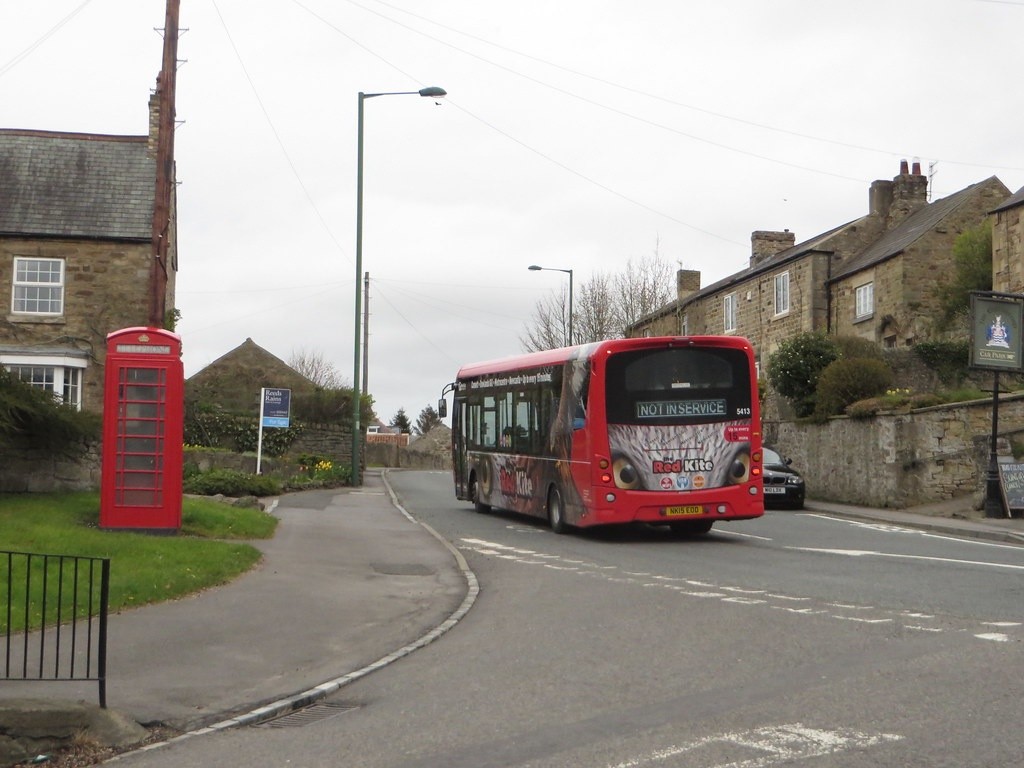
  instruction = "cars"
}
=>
[763,447,805,509]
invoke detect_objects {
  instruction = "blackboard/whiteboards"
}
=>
[998,462,1024,510]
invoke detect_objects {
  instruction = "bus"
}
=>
[439,336,763,536]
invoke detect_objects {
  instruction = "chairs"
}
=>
[517,437,547,457]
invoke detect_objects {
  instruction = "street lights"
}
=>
[352,86,448,489]
[529,265,573,347]
